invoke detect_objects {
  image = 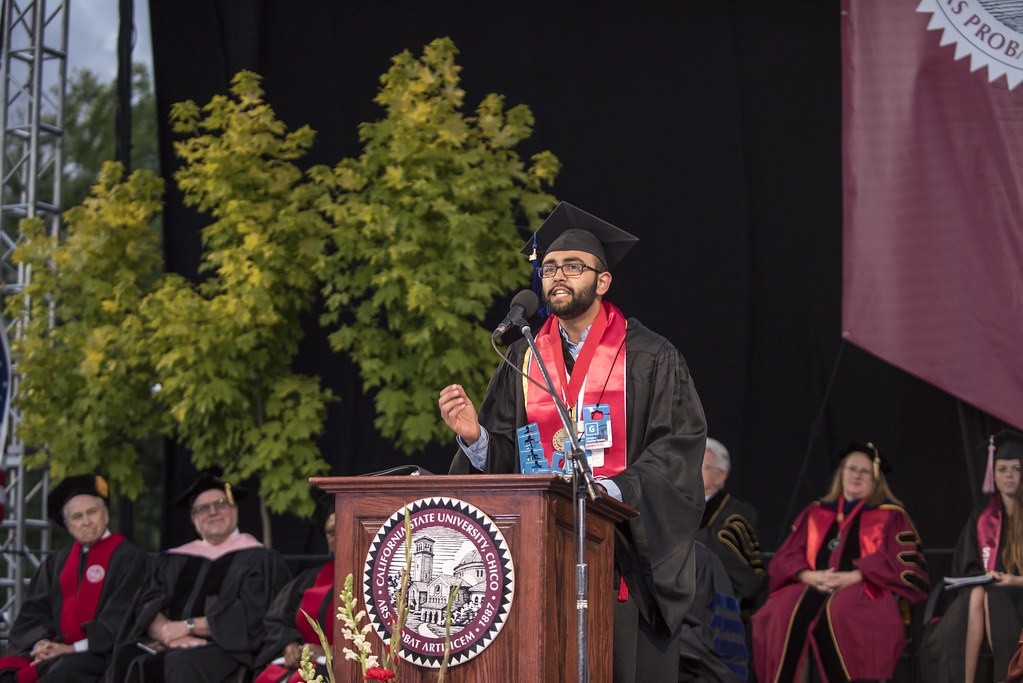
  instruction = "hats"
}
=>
[180,470,250,505]
[520,201,639,318]
[313,495,335,528]
[48,472,110,528]
[978,430,1023,493]
[836,441,892,480]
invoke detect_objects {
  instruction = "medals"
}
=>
[828,539,840,550]
[553,428,569,452]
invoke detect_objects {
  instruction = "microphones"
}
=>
[491,289,539,340]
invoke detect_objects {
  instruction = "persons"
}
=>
[917,435,1023,683]
[0,472,336,683]
[679,436,766,683]
[751,442,931,683]
[440,201,708,683]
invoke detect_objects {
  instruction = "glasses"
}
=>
[192,498,229,514]
[702,463,720,470]
[323,526,335,536]
[536,264,602,278]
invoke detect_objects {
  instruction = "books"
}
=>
[943,573,999,591]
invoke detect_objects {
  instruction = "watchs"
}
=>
[184,618,196,633]
